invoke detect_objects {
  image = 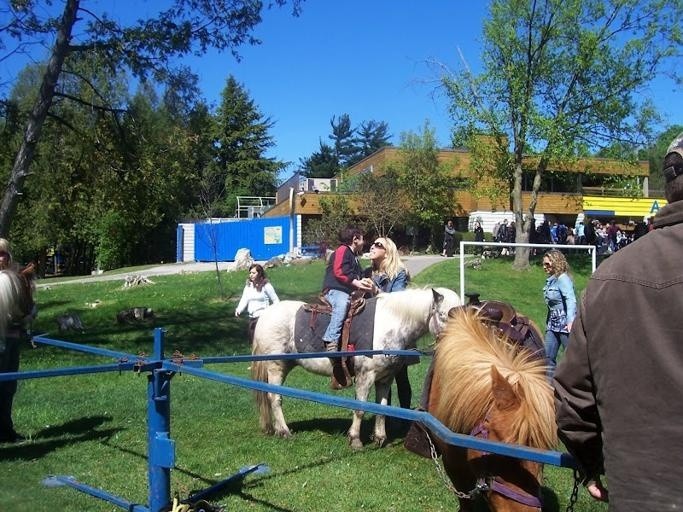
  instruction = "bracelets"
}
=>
[372,286,379,297]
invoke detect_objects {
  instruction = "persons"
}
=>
[0,235,38,446]
[232,263,280,370]
[436,215,654,274]
[318,227,371,359]
[537,249,580,382]
[360,235,415,435]
[548,130,682,511]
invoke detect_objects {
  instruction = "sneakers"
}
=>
[325,341,339,352]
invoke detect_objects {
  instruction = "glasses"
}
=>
[371,242,386,251]
[542,262,552,269]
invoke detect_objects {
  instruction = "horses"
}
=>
[0,261,38,341]
[247,284,463,454]
[420,303,556,511]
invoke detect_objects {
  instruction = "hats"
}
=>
[664,132,682,182]
